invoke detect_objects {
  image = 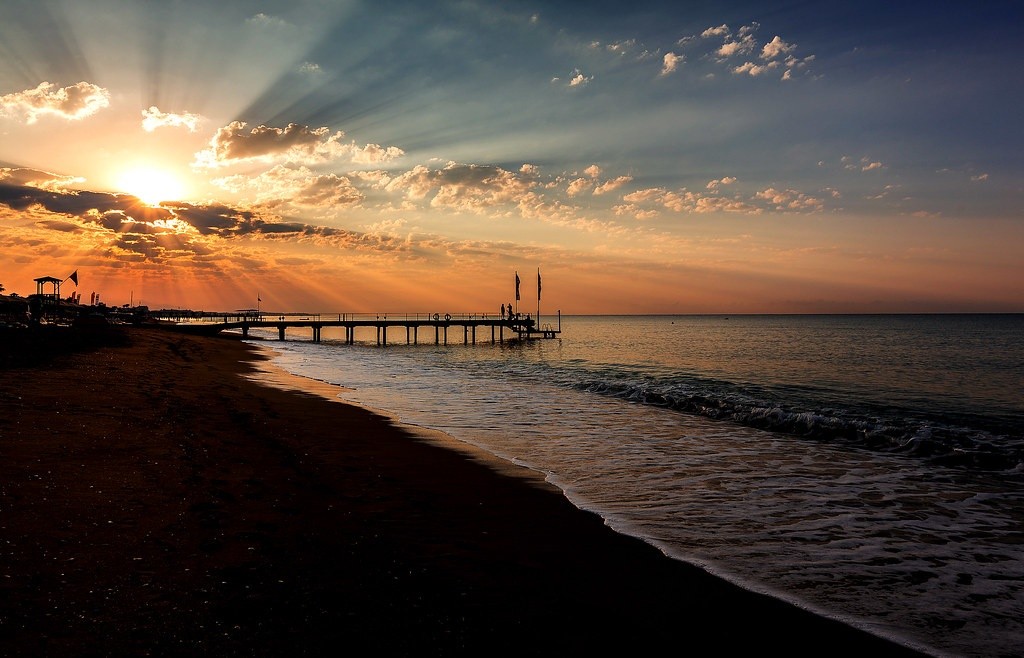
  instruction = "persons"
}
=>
[506,303,512,320]
[501,304,505,320]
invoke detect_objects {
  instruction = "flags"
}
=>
[69,271,78,287]
[258,298,261,301]
[516,274,520,300]
[538,273,541,300]
